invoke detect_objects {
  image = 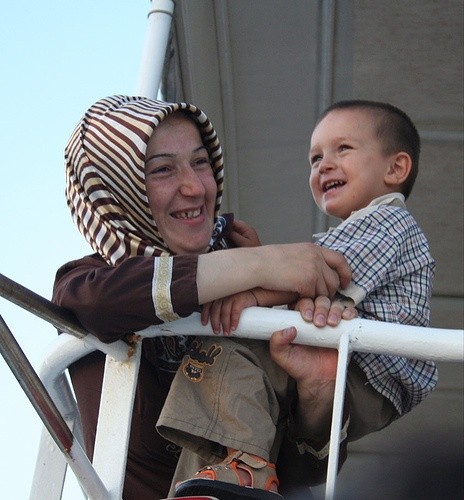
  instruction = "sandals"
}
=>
[174,450,286,499]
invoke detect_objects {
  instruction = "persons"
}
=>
[156,99,439,500]
[52,94,349,500]
